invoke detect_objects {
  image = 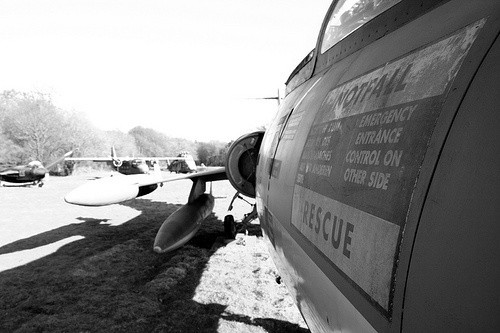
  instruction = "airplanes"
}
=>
[63,0,500,333]
[0,150,72,188]
[64,147,187,171]
